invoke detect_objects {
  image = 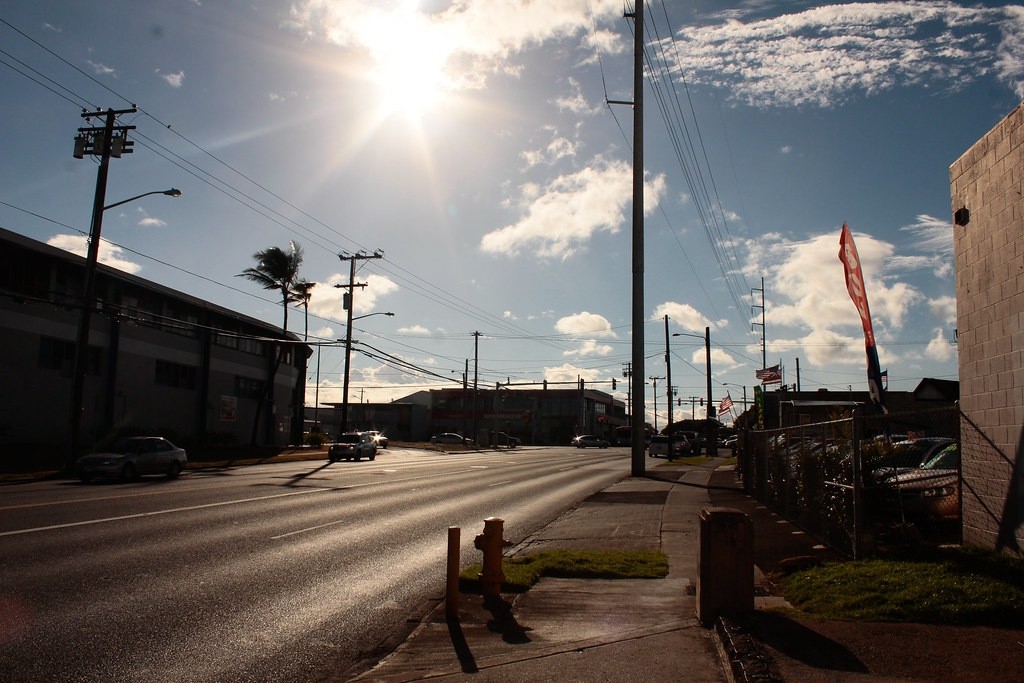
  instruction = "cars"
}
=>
[430,433,474,448]
[673,434,690,455]
[765,434,960,546]
[77,436,188,482]
[571,435,611,449]
[480,431,522,448]
[702,434,739,450]
[649,435,676,456]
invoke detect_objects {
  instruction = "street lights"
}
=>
[451,359,469,445]
[672,327,719,457]
[334,248,395,434]
[68,104,181,477]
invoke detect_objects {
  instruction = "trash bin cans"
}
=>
[695,508,753,628]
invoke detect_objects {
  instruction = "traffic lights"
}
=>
[700,398,703,406]
[678,398,681,406]
[612,377,617,390]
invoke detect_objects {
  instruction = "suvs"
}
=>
[677,431,701,454]
[367,430,388,449]
[328,432,377,462]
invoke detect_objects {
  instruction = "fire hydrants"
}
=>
[473,517,515,583]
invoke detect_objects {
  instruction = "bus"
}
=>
[616,426,651,448]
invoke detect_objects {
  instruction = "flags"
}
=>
[718,408,730,417]
[720,396,733,411]
[880,369,888,382]
[756,365,779,379]
[761,369,782,384]
[838,221,890,421]
